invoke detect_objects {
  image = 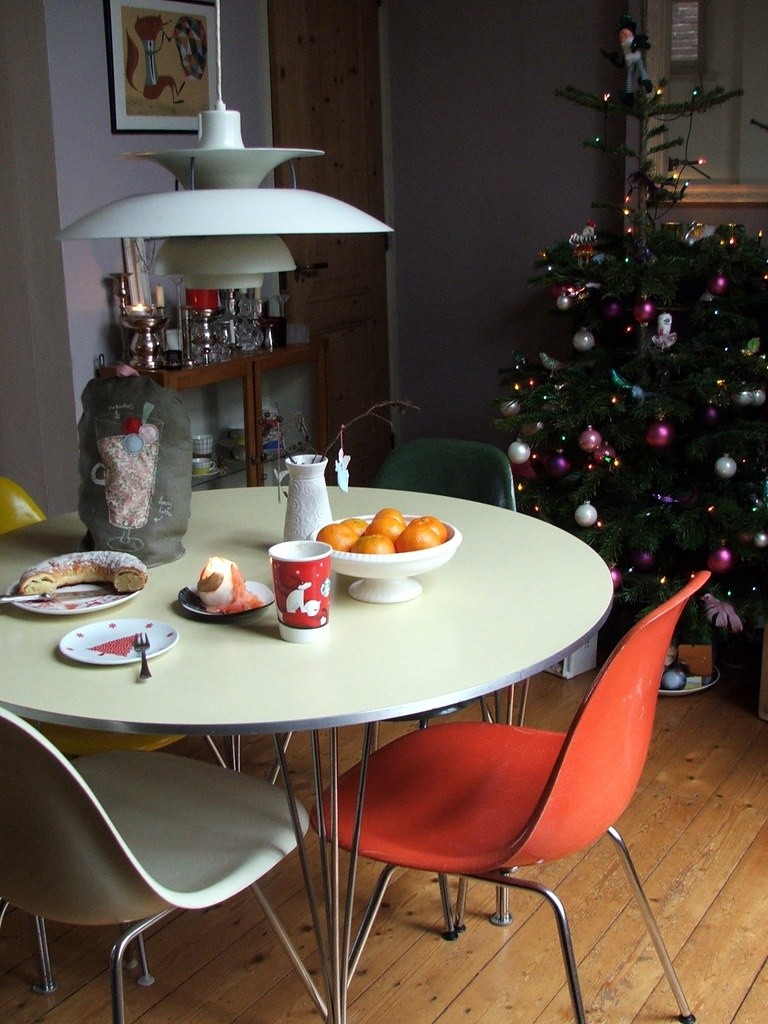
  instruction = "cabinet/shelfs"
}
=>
[100,336,333,491]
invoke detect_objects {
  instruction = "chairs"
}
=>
[0,708,335,1024]
[309,571,713,1024]
[0,478,231,994]
[261,439,519,933]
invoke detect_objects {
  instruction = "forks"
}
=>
[134,632,152,679]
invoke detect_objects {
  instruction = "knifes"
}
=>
[0,587,117,605]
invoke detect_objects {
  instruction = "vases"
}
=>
[283,454,334,543]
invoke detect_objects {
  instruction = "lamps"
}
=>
[56,0,394,290]
[156,283,164,307]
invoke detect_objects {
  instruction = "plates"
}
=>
[307,513,463,605]
[191,468,221,477]
[179,578,275,617]
[58,619,179,665]
[6,574,142,615]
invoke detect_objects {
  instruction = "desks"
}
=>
[1,486,615,1024]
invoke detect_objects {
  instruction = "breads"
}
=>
[20,551,147,595]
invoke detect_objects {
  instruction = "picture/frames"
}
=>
[102,0,221,134]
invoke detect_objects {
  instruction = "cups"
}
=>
[192,433,213,460]
[191,457,216,474]
[268,539,333,644]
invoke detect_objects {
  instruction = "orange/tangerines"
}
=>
[317,507,449,552]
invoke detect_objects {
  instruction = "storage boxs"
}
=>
[544,631,597,679]
